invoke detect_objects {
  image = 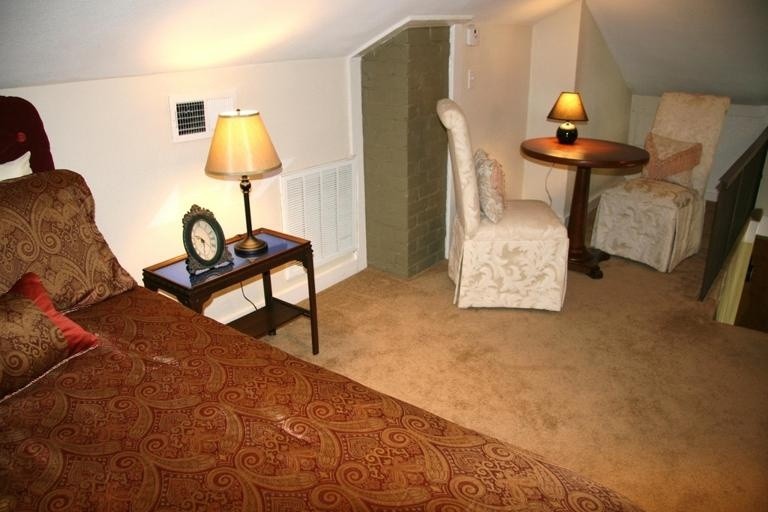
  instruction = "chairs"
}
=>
[436,96,570,314]
[588,90,732,275]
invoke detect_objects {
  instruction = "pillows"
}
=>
[641,131,703,190]
[473,148,507,223]
[0,168,139,313]
[0,273,101,403]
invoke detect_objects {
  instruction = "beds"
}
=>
[0,96,637,512]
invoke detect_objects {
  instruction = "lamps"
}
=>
[547,91,588,144]
[204,109,283,259]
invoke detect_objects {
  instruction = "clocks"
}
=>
[181,204,234,274]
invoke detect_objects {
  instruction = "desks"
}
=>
[519,137,650,279]
[142,227,319,355]
